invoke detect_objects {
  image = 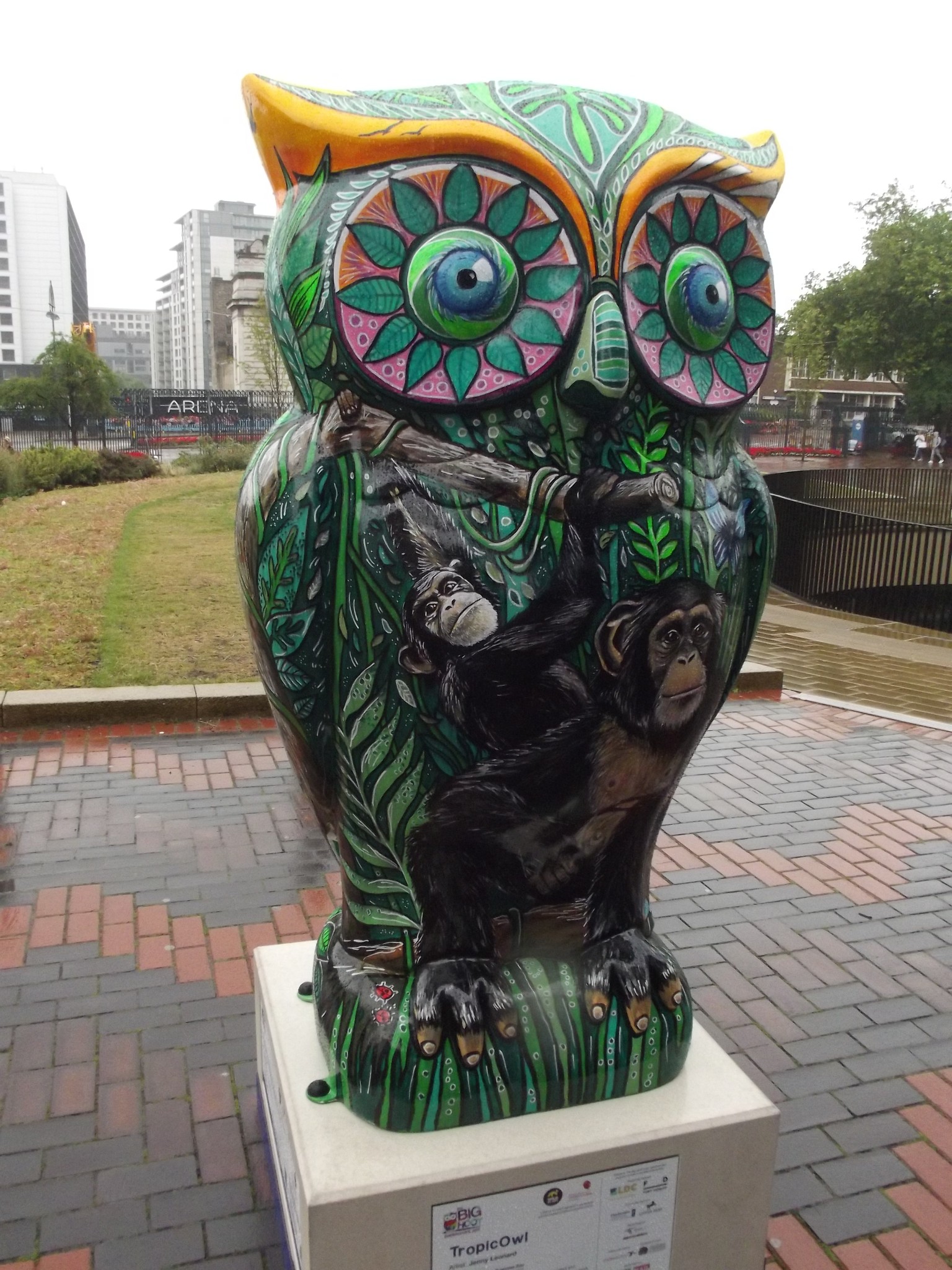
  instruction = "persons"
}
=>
[911,430,926,461]
[927,431,945,464]
[2,437,14,455]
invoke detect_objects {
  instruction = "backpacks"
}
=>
[937,436,947,447]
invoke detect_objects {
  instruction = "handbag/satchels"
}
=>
[919,442,926,449]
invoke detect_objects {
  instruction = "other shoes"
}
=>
[912,457,915,461]
[928,460,933,463]
[938,460,944,463]
[917,459,922,461]
